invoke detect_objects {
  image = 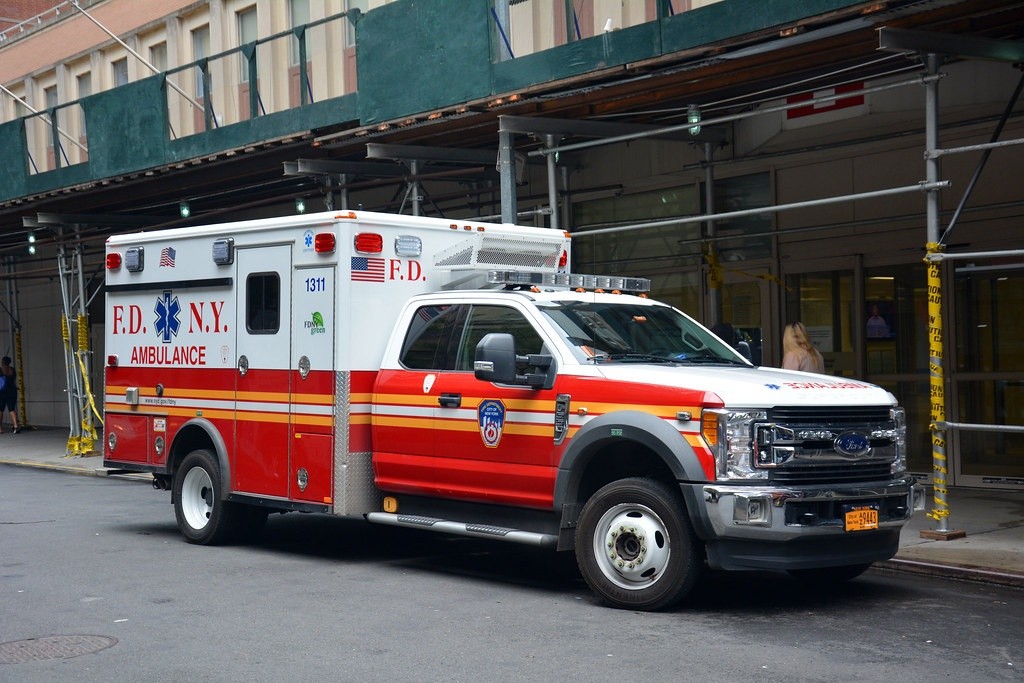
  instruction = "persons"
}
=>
[0,357,20,434]
[866,305,891,337]
[782,322,825,375]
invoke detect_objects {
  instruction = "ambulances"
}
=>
[96,209,929,614]
